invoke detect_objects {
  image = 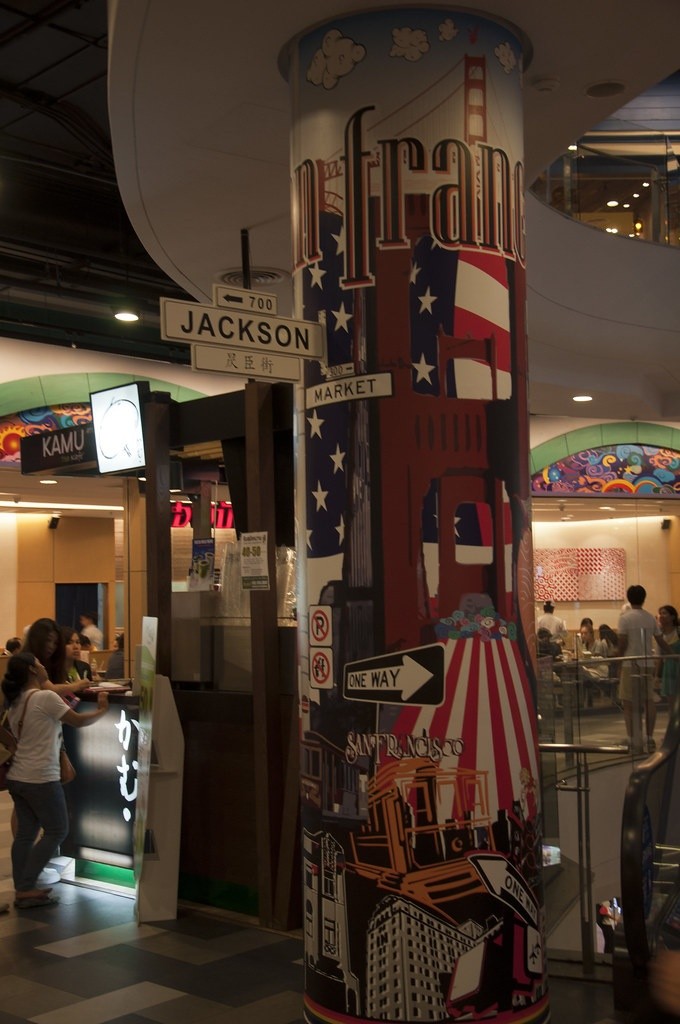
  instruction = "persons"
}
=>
[4,618,90,885]
[600,624,618,658]
[580,617,594,632]
[2,651,109,910]
[572,625,609,677]
[4,610,124,682]
[656,605,680,740]
[596,901,621,953]
[614,585,678,748]
[536,599,567,654]
[537,626,585,686]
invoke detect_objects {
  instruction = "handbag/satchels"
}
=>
[59,746,77,786]
[0,755,11,793]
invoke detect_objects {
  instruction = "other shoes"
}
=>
[13,886,60,908]
[35,867,60,885]
[614,738,657,754]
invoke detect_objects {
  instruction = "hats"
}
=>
[544,598,557,607]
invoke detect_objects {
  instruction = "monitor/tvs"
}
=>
[89,380,151,477]
